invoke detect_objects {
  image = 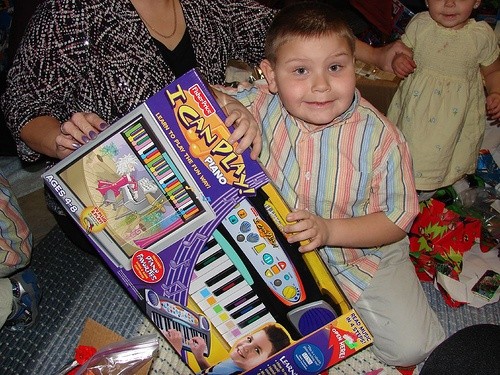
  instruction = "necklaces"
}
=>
[137,0,177,38]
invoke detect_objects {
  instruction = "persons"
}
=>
[0,0,416,331]
[208,2,445,367]
[161,324,290,375]
[386,0,500,193]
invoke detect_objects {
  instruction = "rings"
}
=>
[60,121,69,136]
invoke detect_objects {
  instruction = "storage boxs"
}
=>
[42,68,375,375]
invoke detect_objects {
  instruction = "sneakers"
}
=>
[8,268,41,331]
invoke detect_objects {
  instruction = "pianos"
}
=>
[139,287,211,357]
[188,188,343,370]
